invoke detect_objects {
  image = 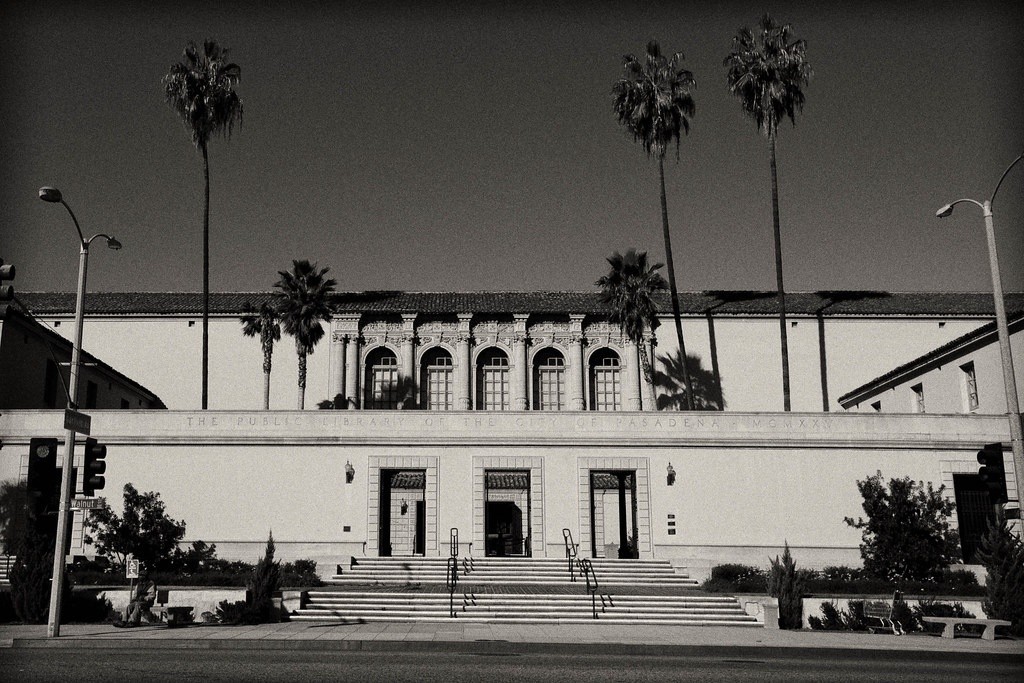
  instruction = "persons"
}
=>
[113,571,156,628]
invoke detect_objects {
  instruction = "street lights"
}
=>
[37,185,125,638]
[936,151,1024,540]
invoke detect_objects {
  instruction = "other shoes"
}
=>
[124,622,140,627]
[113,620,124,627]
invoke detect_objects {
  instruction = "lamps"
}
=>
[345,460,353,483]
[400,498,406,514]
[666,461,676,485]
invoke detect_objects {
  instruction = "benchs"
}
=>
[923,616,1011,641]
[116,606,194,626]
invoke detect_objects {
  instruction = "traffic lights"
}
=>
[977,442,1008,506]
[83,436,106,496]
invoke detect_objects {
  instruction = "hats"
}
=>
[139,570,147,575]
